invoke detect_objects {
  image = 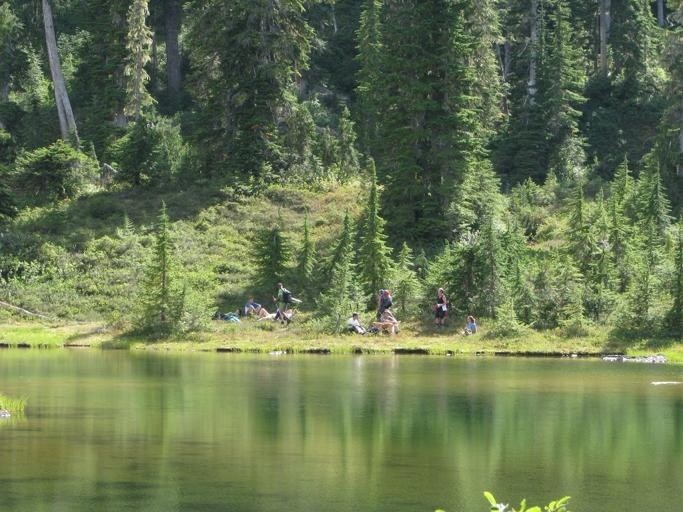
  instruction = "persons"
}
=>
[210,308,241,319]
[431,287,449,330]
[270,281,291,321]
[257,307,291,320]
[371,288,400,334]
[243,296,269,317]
[345,311,366,335]
[456,315,476,338]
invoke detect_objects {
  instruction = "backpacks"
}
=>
[282,288,291,303]
